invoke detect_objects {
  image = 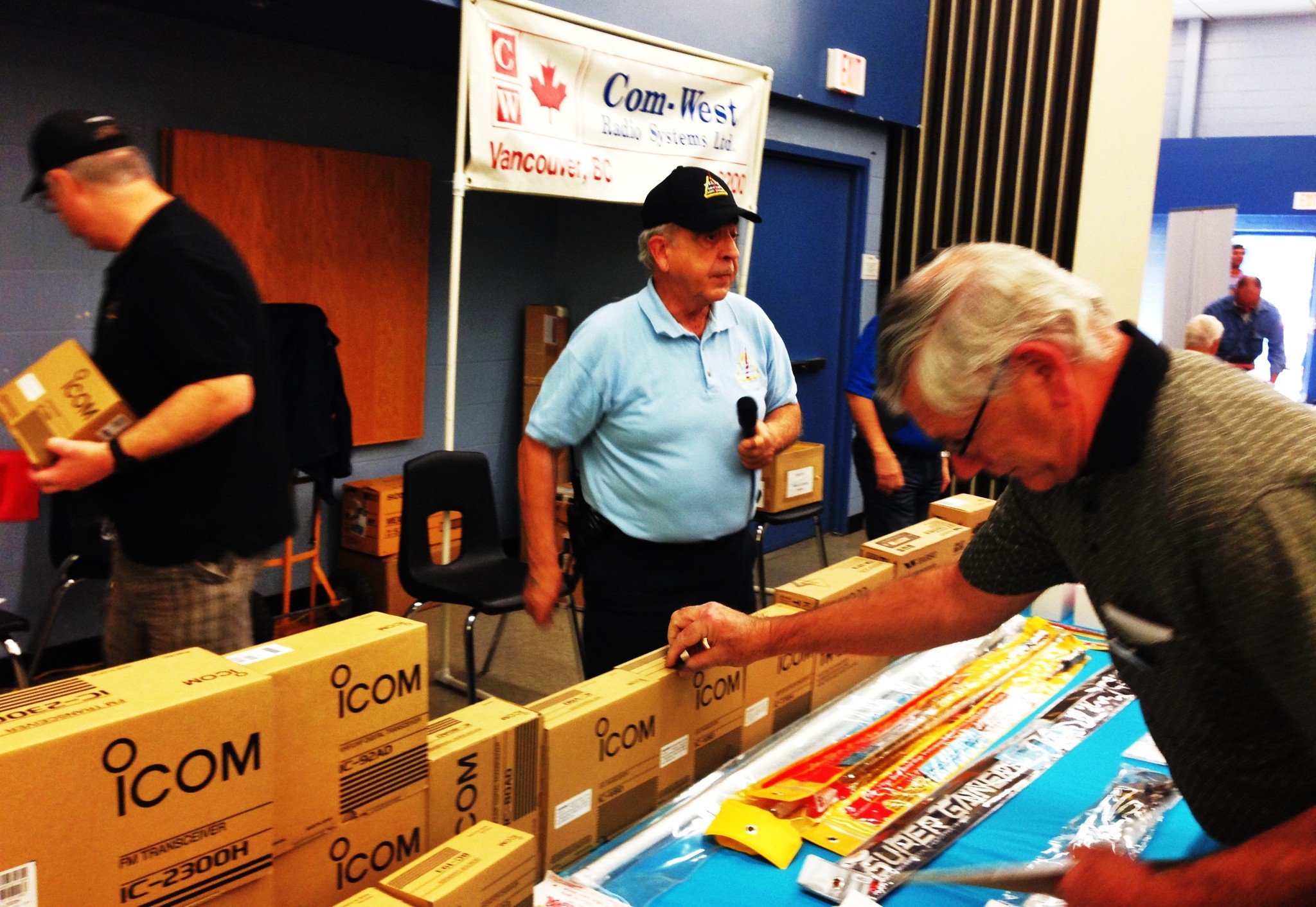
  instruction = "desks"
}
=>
[535,606,1215,905]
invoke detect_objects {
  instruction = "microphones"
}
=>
[736,396,758,482]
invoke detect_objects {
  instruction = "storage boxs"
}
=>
[338,472,465,626]
[756,438,829,507]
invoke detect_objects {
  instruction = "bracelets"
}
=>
[109,437,140,469]
[940,451,951,458]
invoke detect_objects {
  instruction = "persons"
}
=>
[1227,243,1247,294]
[1202,275,1289,386]
[17,99,304,657]
[665,237,1316,907]
[1183,313,1226,356]
[514,165,804,680]
[842,247,952,541]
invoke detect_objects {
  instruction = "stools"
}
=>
[749,501,835,608]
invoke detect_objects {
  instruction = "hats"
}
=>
[638,165,763,231]
[20,108,136,203]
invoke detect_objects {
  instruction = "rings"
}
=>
[748,437,757,449]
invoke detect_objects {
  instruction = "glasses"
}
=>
[38,189,60,215]
[931,355,1010,461]
